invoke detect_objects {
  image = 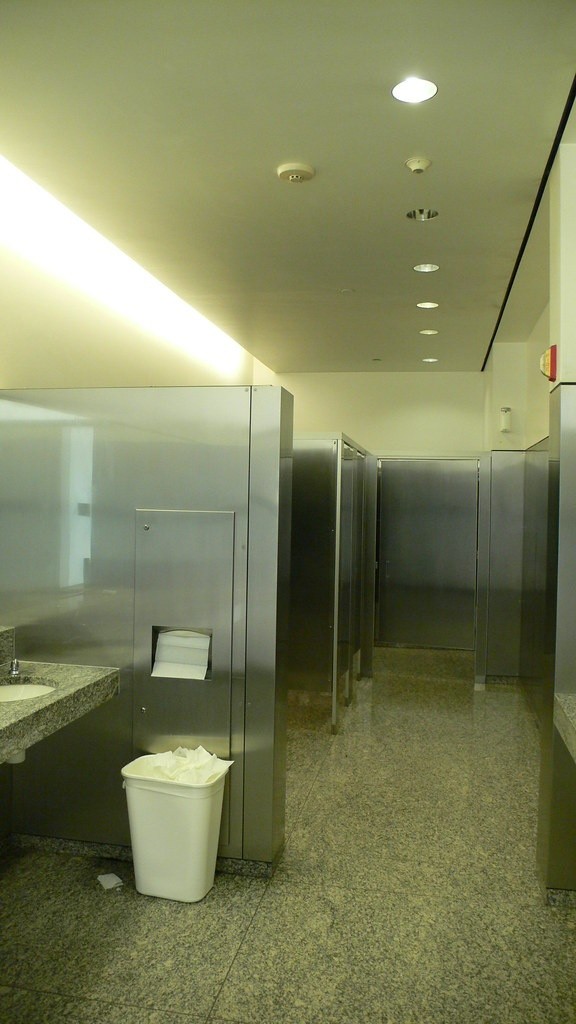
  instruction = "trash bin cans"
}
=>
[120,752,230,902]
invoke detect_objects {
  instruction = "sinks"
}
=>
[0,676,59,702]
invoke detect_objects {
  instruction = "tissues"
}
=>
[150,625,213,682]
